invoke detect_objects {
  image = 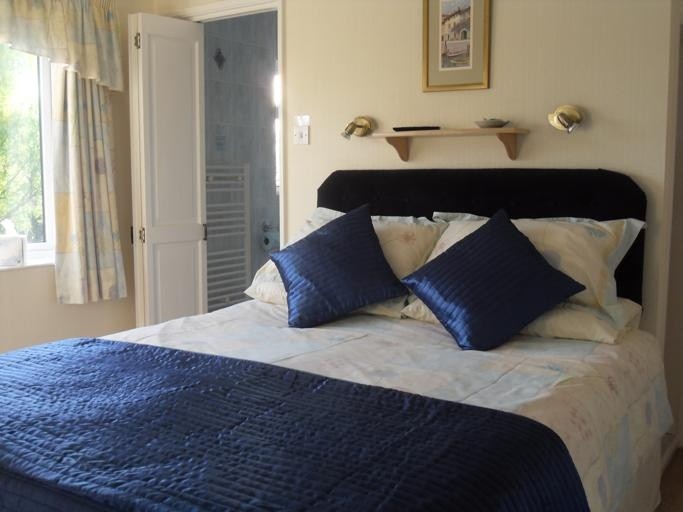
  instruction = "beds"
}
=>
[0,166,670,511]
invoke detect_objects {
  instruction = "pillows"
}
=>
[400,212,588,351]
[425,218,646,333]
[268,206,409,329]
[282,208,448,279]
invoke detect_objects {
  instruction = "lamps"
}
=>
[547,102,586,136]
[339,116,374,142]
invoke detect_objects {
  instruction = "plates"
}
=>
[474,120,510,128]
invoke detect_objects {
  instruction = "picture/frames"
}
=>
[419,0,491,95]
